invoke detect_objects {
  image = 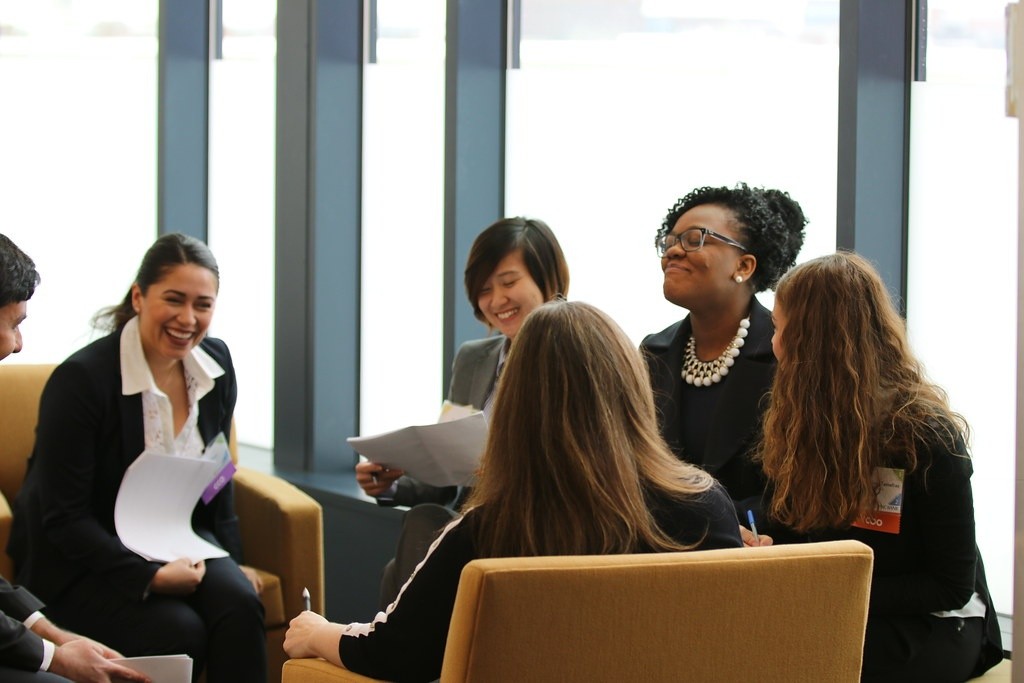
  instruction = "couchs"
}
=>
[281,539,874,683]
[0,362,327,683]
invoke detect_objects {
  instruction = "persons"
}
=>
[281,303,742,683]
[7,232,270,683]
[740,253,1003,683]
[638,185,806,501]
[0,233,150,683]
[355,217,569,613]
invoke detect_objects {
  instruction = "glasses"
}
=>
[655,226,746,258]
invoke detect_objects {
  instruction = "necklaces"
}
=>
[681,314,752,387]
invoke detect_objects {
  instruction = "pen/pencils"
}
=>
[747,509,759,541]
[303,586,311,610]
